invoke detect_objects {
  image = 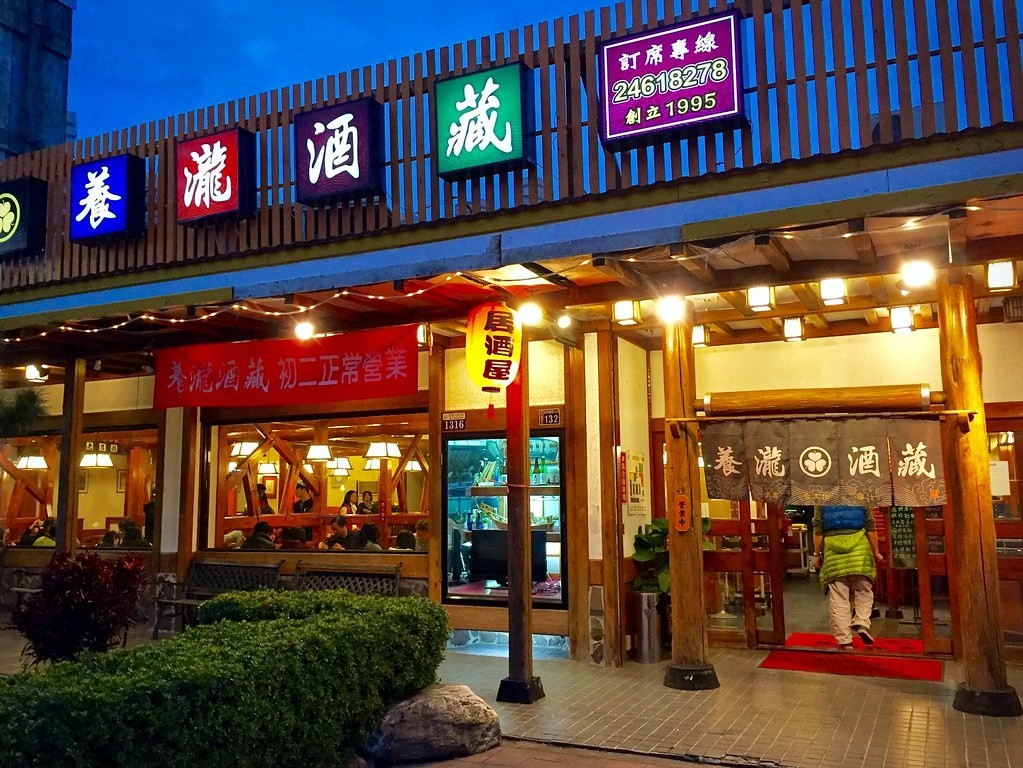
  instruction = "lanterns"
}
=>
[465,303,522,418]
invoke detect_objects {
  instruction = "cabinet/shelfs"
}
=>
[471,486,560,542]
[721,524,811,612]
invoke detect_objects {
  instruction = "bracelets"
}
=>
[814,552,821,555]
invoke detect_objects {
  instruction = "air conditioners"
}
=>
[868,100,945,145]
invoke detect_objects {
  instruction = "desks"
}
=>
[448,578,561,606]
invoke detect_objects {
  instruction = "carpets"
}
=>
[757,649,944,682]
[785,632,926,655]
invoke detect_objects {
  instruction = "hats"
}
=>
[297,478,307,487]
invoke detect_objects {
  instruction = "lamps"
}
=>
[227,436,421,476]
[545,309,571,330]
[79,453,115,471]
[94,360,102,370]
[996,432,1015,452]
[24,361,49,384]
[610,256,1023,349]
[293,315,314,340]
[141,355,155,372]
[16,436,50,470]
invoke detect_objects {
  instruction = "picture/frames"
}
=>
[117,469,127,492]
[78,468,89,494]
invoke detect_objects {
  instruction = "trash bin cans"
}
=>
[639,593,662,663]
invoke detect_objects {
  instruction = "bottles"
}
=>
[483,456,550,486]
[462,507,498,530]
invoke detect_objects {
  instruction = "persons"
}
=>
[350,524,382,550]
[389,529,416,551]
[0,517,57,546]
[812,506,883,650]
[338,490,360,531]
[224,480,313,551]
[319,515,352,550]
[356,491,378,514]
[97,484,156,550]
[372,501,394,514]
[412,519,429,550]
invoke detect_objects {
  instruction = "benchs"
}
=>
[147,552,285,639]
[8,569,43,621]
[294,558,403,599]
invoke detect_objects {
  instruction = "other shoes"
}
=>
[857,627,874,645]
[840,642,853,650]
